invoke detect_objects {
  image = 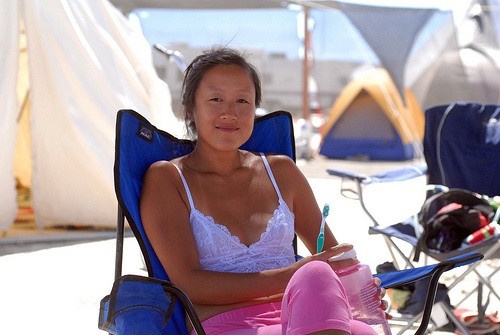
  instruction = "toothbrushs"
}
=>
[316,201,330,253]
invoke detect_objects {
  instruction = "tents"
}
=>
[0,0,189,234]
[314,63,425,160]
[402,4,499,109]
[129,6,388,126]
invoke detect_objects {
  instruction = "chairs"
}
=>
[325,101,499,332]
[98,109,307,335]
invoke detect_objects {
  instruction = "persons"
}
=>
[140,46,393,335]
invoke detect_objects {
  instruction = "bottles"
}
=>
[327,247,393,335]
